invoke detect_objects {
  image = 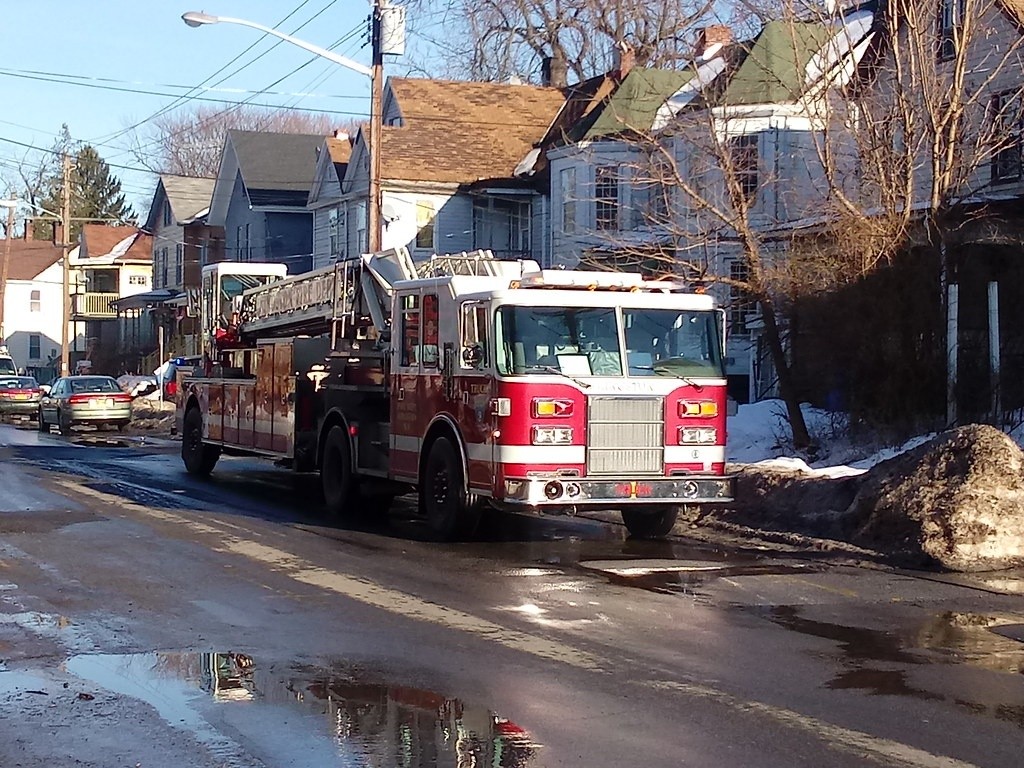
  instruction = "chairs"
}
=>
[627,352,655,376]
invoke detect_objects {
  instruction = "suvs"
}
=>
[164,355,205,403]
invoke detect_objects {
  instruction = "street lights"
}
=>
[0,197,70,375]
[182,12,382,254]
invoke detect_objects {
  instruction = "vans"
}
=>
[0,354,19,376]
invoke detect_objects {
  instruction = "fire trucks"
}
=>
[167,242,734,540]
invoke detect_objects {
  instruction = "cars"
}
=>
[38,375,133,435]
[0,375,45,424]
